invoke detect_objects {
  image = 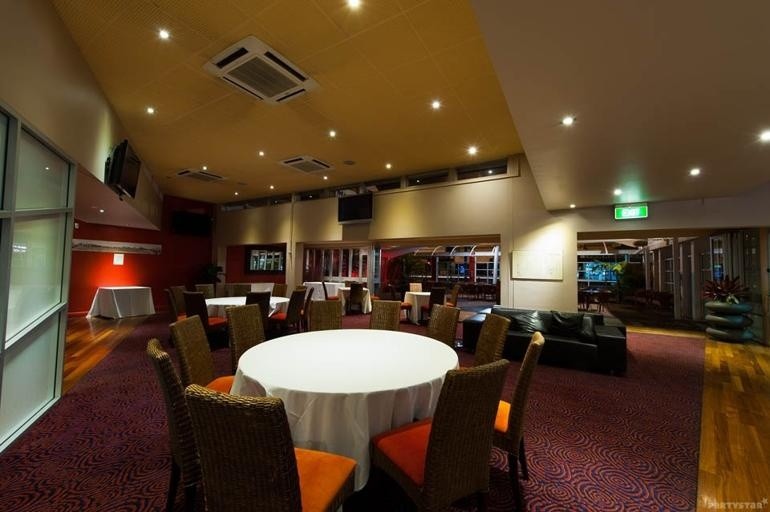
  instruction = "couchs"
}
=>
[462,304,628,378]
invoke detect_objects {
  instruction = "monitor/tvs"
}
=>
[172,210,212,239]
[337,192,373,224]
[108,138,142,200]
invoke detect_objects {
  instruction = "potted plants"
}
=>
[700,274,755,342]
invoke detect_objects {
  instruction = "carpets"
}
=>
[0,313,705,512]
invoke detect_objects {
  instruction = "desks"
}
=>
[85,286,156,321]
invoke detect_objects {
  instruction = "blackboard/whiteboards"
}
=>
[511,249,564,281]
